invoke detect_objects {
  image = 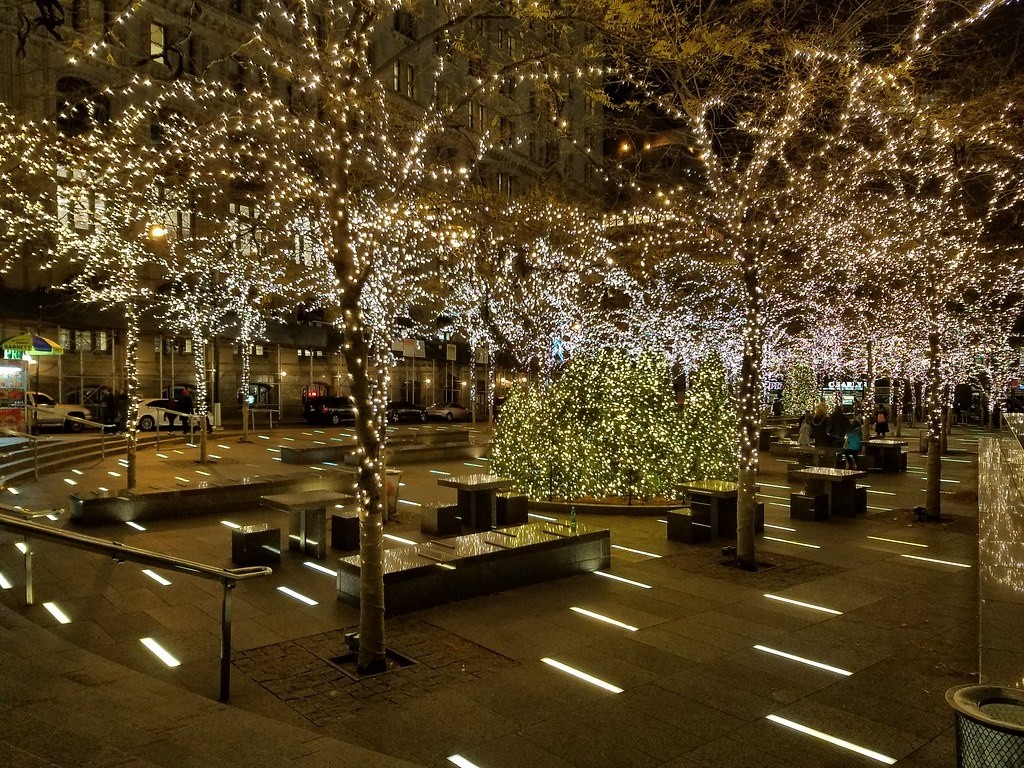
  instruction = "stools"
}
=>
[231,523,281,567]
[856,484,867,513]
[496,491,528,525]
[421,498,461,537]
[666,508,710,545]
[754,502,764,534]
[790,491,828,522]
[770,436,779,442]
[788,464,810,483]
[858,455,875,469]
[901,450,908,472]
[331,512,360,552]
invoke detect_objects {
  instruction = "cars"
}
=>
[304,395,357,426]
[26,390,92,433]
[424,402,472,421]
[133,398,214,432]
[386,399,426,424]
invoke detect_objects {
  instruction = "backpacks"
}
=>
[876,412,886,423]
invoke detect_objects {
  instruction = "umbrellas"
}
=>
[1,331,64,356]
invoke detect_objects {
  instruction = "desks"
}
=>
[760,427,777,452]
[674,480,760,538]
[860,439,908,472]
[790,446,842,469]
[793,467,867,519]
[259,489,354,560]
[436,473,517,531]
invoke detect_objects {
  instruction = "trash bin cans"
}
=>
[946,683,1024,768]
[919,429,928,452]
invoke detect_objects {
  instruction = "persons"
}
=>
[800,405,862,471]
[6,388,22,407]
[195,407,213,434]
[163,398,178,435]
[117,388,131,435]
[177,389,194,434]
[102,388,118,436]
[874,403,889,436]
